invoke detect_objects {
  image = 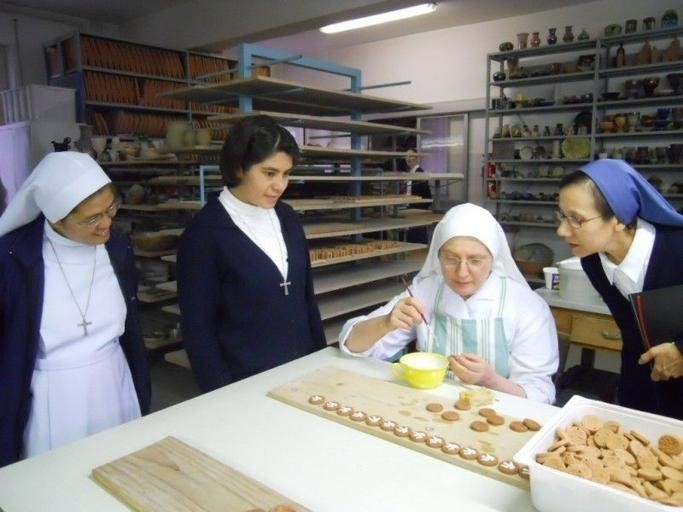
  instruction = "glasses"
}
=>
[554,209,602,228]
[76,197,122,226]
[440,255,487,270]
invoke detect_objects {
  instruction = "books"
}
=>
[628,285,682,372]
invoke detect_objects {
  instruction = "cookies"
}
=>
[536,415,683,507]
[524,419,540,431]
[309,395,529,480]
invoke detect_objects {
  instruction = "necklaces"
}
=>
[46,237,98,336]
[233,208,292,296]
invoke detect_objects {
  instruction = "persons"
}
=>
[403,147,432,244]
[176,115,327,392]
[0,151,153,467]
[556,158,683,420]
[337,202,560,405]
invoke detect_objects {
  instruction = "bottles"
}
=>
[502,122,568,138]
[167,123,214,152]
[513,9,680,75]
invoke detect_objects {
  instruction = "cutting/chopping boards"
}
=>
[266,365,551,489]
[91,435,312,511]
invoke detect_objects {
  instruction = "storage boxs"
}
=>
[555,256,606,306]
[0,85,78,123]
[513,393,683,511]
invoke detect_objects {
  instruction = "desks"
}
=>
[533,284,624,374]
[0,348,559,511]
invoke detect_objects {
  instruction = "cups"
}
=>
[499,166,568,201]
[389,351,449,390]
[542,267,560,291]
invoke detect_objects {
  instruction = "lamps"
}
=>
[319,0,439,35]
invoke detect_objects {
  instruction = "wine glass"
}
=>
[89,134,165,165]
[596,74,682,164]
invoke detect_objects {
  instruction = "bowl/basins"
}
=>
[141,332,166,344]
[491,93,592,108]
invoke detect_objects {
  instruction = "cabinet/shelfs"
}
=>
[154,39,466,370]
[482,25,683,285]
[45,28,270,305]
